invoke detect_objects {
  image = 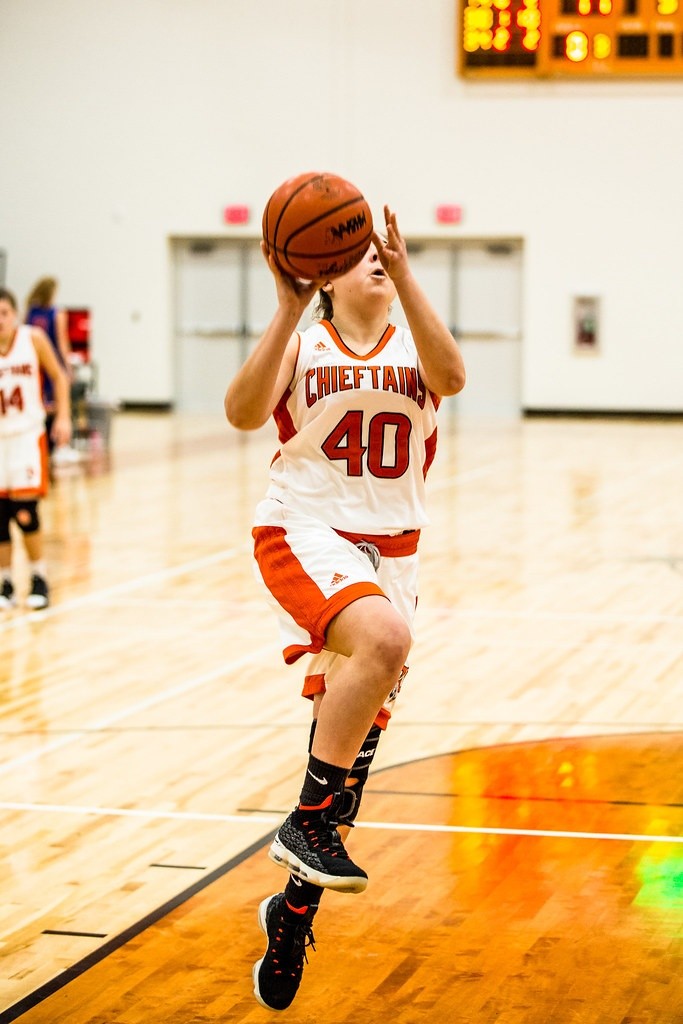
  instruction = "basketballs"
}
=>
[261,172,374,280]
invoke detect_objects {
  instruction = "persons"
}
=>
[24,277,71,486]
[0,287,71,613]
[224,205,468,1013]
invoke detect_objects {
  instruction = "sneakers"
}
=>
[0,581,16,611]
[26,574,49,608]
[252,889,309,1009]
[267,812,371,892]
